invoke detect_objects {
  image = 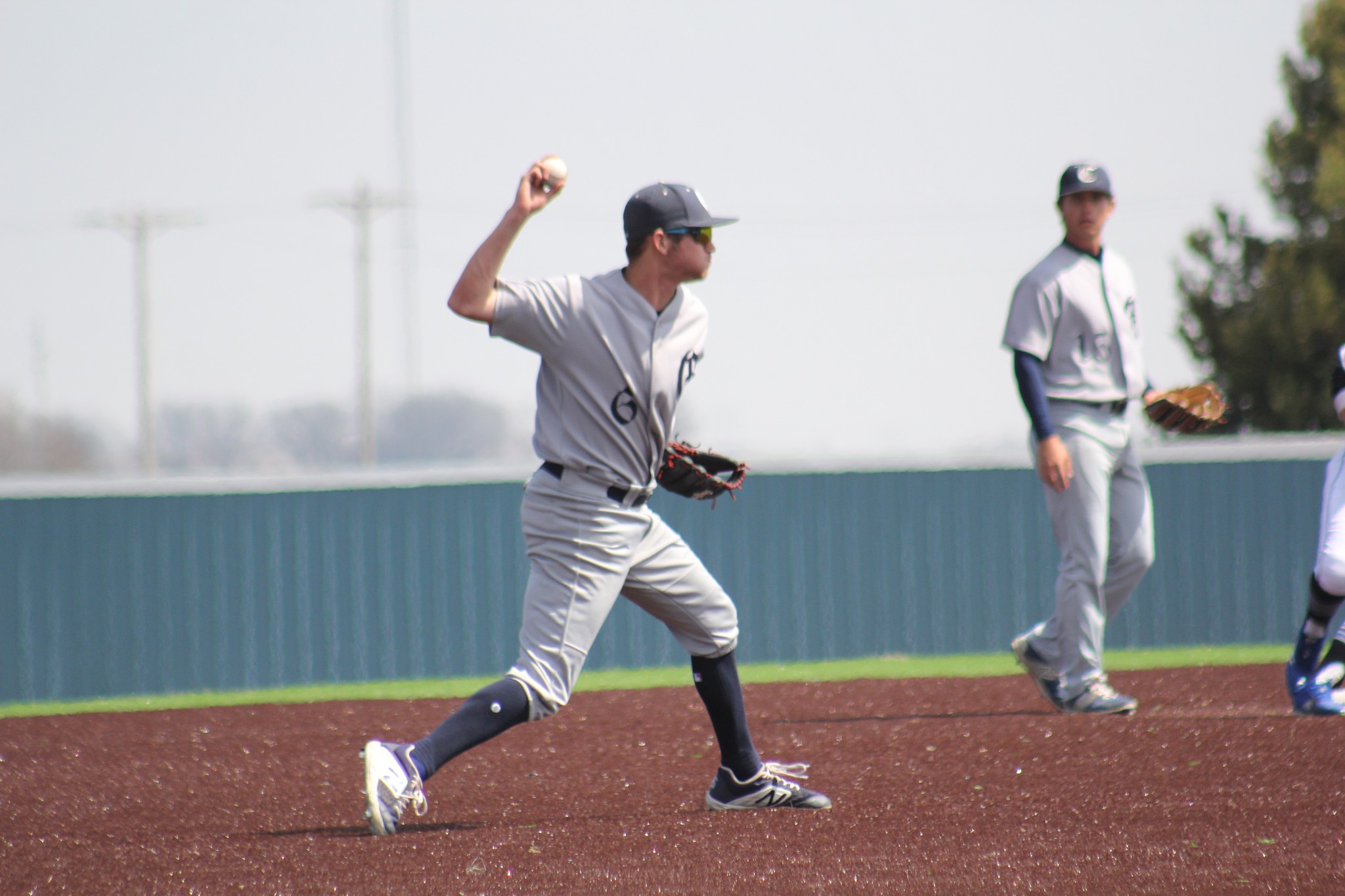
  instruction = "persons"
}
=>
[1001,162,1226,716]
[1284,344,1345,717]
[362,154,834,835]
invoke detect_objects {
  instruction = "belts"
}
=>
[1048,396,1128,415]
[542,460,646,507]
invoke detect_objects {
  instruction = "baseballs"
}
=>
[541,153,569,188]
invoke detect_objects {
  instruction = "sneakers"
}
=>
[1010,632,1070,713]
[364,738,423,835]
[1286,660,1345,716]
[1071,682,1137,714]
[704,765,833,811]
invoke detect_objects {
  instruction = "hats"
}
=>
[624,183,740,233]
[1060,164,1112,197]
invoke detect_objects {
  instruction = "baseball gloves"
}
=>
[1139,382,1233,440]
[656,443,747,501]
